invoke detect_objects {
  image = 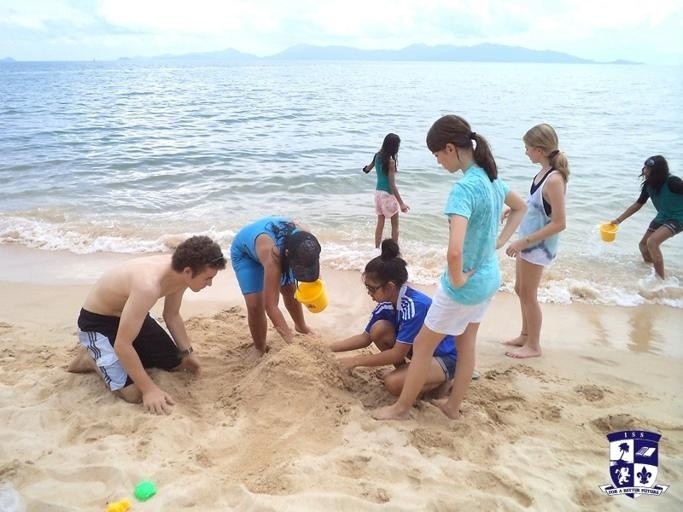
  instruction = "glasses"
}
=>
[365,281,387,292]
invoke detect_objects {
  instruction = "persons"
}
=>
[497,122,570,359]
[610,155,683,280]
[230,214,323,354]
[365,113,530,420]
[361,132,411,249]
[326,239,457,402]
[60,234,227,415]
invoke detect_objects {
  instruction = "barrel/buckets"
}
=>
[599,221,620,242]
[289,278,331,315]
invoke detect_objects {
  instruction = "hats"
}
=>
[288,231,321,282]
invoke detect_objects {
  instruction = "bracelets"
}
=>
[615,216,622,224]
[181,345,195,356]
[524,237,530,244]
